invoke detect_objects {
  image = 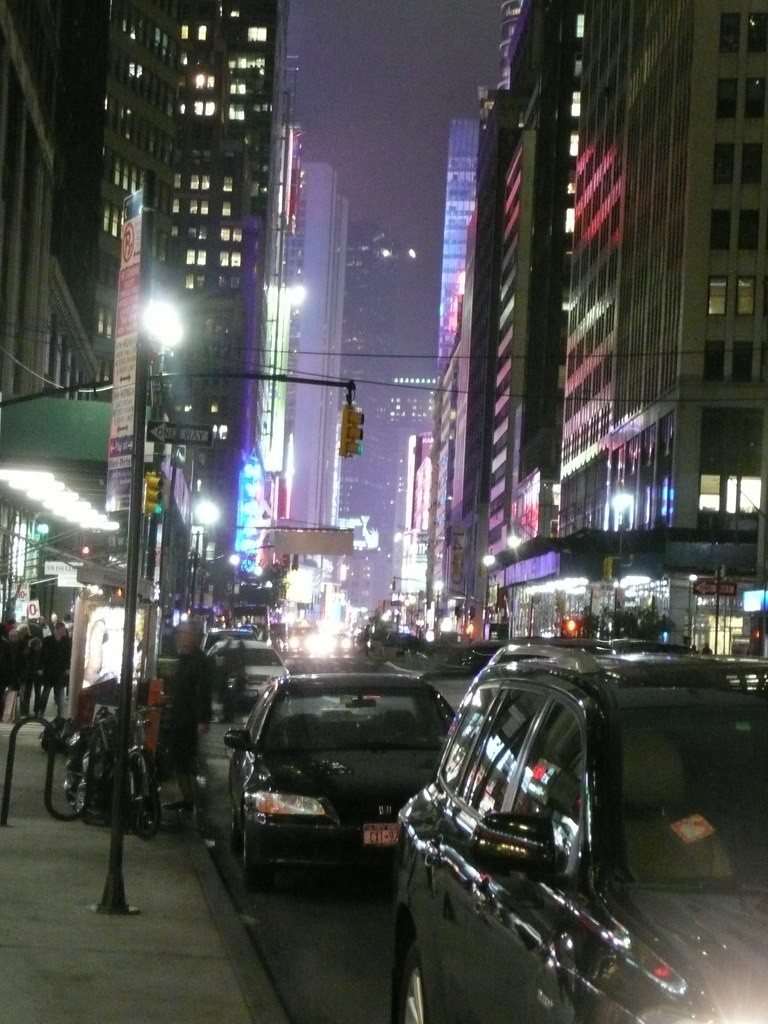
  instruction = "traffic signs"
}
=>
[146,419,213,448]
[692,579,738,597]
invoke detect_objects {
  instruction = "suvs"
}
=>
[389,643,768,1024]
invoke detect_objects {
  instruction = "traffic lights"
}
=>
[602,557,620,582]
[562,620,581,632]
[143,474,164,516]
[338,405,366,460]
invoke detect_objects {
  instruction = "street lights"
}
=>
[506,522,523,640]
[125,299,190,713]
[482,547,497,641]
[189,500,219,620]
[227,554,239,627]
[687,570,698,639]
[612,491,634,639]
[253,565,264,626]
[433,581,443,643]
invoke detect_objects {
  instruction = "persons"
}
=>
[624,611,638,637]
[692,643,713,655]
[0,616,72,724]
[166,620,211,814]
[362,625,386,658]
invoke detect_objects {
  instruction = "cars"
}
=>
[201,623,265,654]
[205,641,292,702]
[223,670,459,891]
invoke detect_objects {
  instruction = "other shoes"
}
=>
[162,799,194,812]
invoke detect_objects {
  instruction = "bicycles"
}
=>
[61,706,164,841]
